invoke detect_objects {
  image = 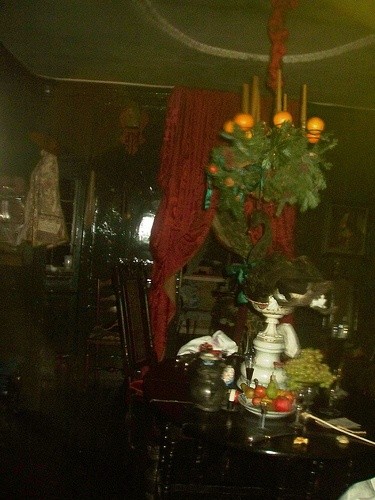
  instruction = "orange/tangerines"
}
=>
[223,112,325,143]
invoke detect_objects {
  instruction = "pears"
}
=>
[265,375,277,399]
[240,382,255,399]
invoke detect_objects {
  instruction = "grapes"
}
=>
[283,347,337,390]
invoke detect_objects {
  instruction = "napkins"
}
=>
[328,417,361,429]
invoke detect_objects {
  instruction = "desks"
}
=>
[143,359,375,500]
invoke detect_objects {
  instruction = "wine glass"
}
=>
[318,378,342,416]
[289,389,308,429]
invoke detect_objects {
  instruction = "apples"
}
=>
[252,385,294,411]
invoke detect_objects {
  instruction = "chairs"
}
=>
[111,259,165,454]
[85,276,123,376]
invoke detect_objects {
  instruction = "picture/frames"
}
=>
[320,199,368,260]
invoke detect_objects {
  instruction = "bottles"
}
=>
[175,343,239,412]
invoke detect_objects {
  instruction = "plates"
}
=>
[238,391,296,418]
